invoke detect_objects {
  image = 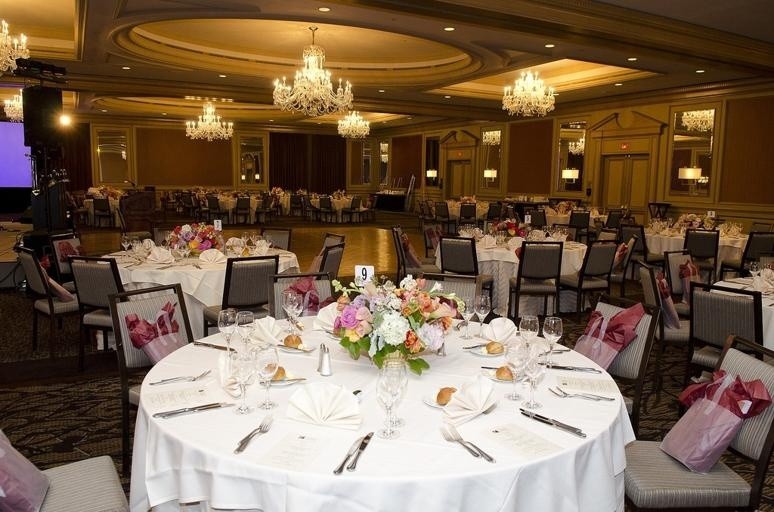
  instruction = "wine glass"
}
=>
[473,296,492,340]
[747,261,774,287]
[280,289,305,337]
[216,309,281,416]
[456,295,476,341]
[456,223,571,246]
[649,216,743,239]
[121,230,272,266]
[375,359,410,440]
[504,313,565,409]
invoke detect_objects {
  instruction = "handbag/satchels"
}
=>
[47,277,75,304]
[574,334,618,369]
[613,251,623,270]
[661,294,682,331]
[683,274,701,305]
[0,431,50,511]
[658,397,745,475]
[142,331,190,364]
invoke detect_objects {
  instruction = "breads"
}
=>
[265,363,285,380]
[486,341,504,354]
[495,366,513,380]
[284,335,302,348]
[437,387,457,405]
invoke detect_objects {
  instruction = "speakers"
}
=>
[23,232,81,292]
[22,86,64,160]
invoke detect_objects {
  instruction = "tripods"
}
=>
[0,151,64,287]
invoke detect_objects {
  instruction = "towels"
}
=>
[443,379,501,426]
[218,350,251,397]
[283,380,363,431]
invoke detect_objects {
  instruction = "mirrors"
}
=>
[663,101,720,204]
[554,118,586,196]
[479,129,502,193]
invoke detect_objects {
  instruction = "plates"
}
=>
[279,342,313,355]
[483,364,523,383]
[423,394,445,410]
[468,343,503,357]
[260,366,303,388]
[322,329,343,341]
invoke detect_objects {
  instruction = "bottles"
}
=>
[316,343,332,377]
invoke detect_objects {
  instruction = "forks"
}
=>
[148,369,211,386]
[440,427,480,458]
[547,386,615,403]
[450,426,493,462]
[233,416,273,455]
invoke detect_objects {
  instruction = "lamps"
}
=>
[338,112,370,140]
[184,102,233,142]
[560,132,587,185]
[482,132,501,182]
[4,87,23,124]
[501,69,558,118]
[0,19,30,73]
[270,27,353,118]
[677,111,715,196]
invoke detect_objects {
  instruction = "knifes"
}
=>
[194,341,237,354]
[347,431,374,473]
[517,405,587,439]
[545,363,602,374]
[332,435,364,476]
[152,402,234,419]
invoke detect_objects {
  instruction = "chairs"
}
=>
[678,280,774,415]
[104,283,194,475]
[624,333,774,512]
[0,424,129,512]
[576,294,662,440]
[15,185,372,374]
[381,192,752,345]
